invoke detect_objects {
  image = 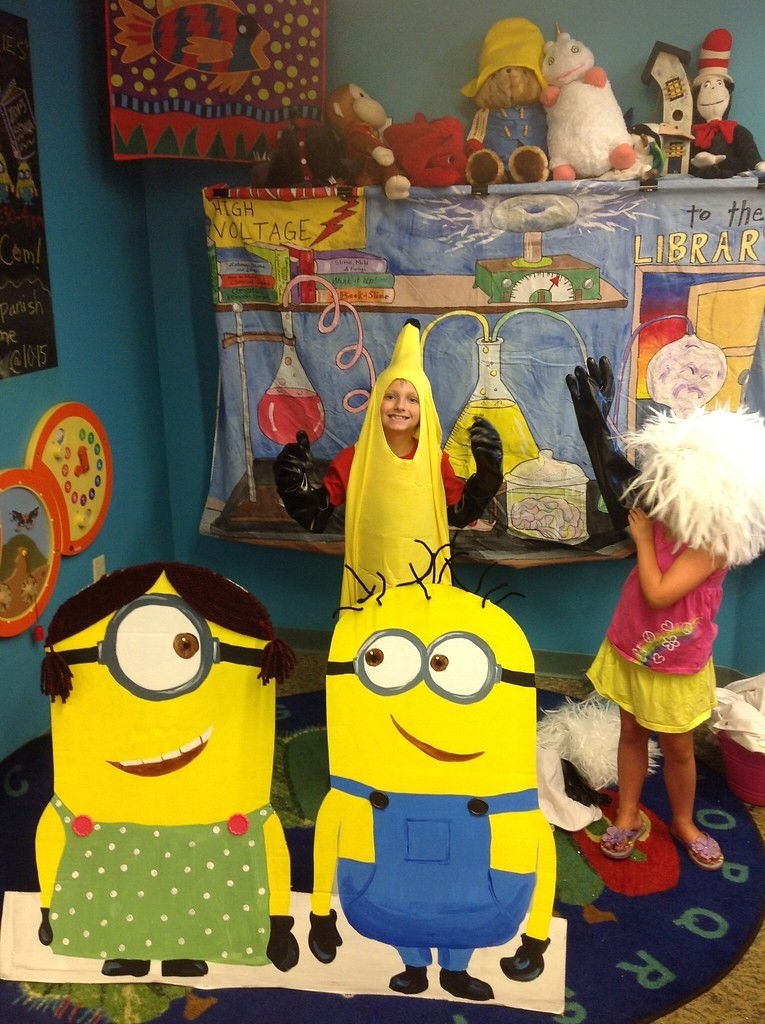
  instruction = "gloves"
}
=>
[272,430,336,533]
[566,355,659,533]
[448,416,504,527]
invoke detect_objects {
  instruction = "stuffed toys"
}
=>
[251,84,485,199]
[461,16,549,185]
[540,32,635,181]
[691,28,765,179]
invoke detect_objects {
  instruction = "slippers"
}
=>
[601,824,646,858]
[671,827,723,870]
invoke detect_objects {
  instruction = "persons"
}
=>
[565,357,765,870]
[273,318,503,610]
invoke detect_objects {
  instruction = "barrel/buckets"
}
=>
[718,731,765,807]
